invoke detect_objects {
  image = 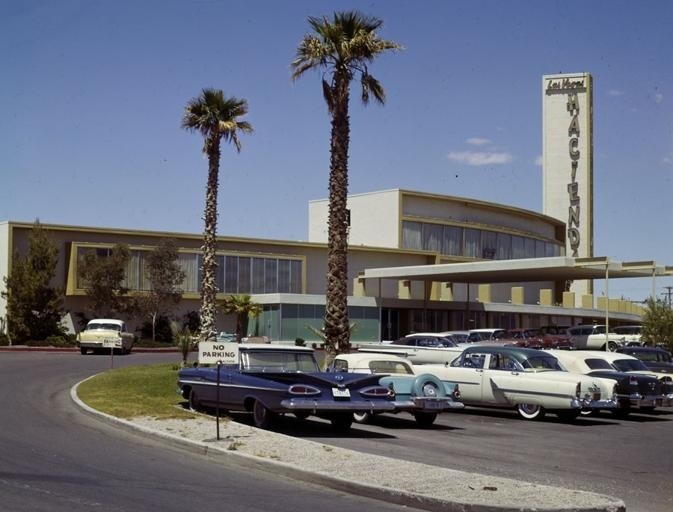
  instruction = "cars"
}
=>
[382,322,673,418]
[176,341,396,431]
[75,317,136,356]
[321,352,464,429]
